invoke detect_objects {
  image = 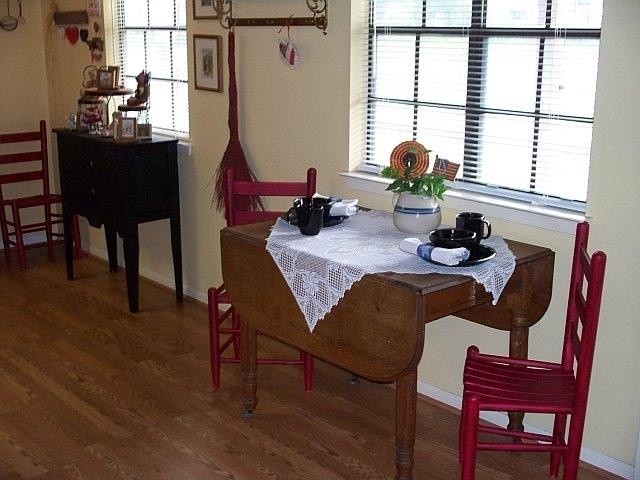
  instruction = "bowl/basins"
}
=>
[293,195,334,216]
[428,227,480,252]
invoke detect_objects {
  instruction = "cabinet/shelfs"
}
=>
[51,123,183,314]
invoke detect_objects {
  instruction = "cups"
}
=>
[286,199,324,237]
[456,211,493,243]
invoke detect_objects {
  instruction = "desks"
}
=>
[221,206,556,480]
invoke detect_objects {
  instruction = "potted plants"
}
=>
[382,143,447,235]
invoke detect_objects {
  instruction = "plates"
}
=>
[280,210,349,228]
[417,241,496,268]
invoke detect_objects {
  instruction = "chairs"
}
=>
[0,120,83,271]
[458,219,608,479]
[206,169,318,394]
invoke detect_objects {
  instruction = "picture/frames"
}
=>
[118,117,137,141]
[107,65,119,87]
[193,33,223,93]
[193,0,223,21]
[97,69,116,91]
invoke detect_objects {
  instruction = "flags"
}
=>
[433,158,461,182]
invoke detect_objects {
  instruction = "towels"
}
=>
[311,193,358,217]
[399,237,468,265]
